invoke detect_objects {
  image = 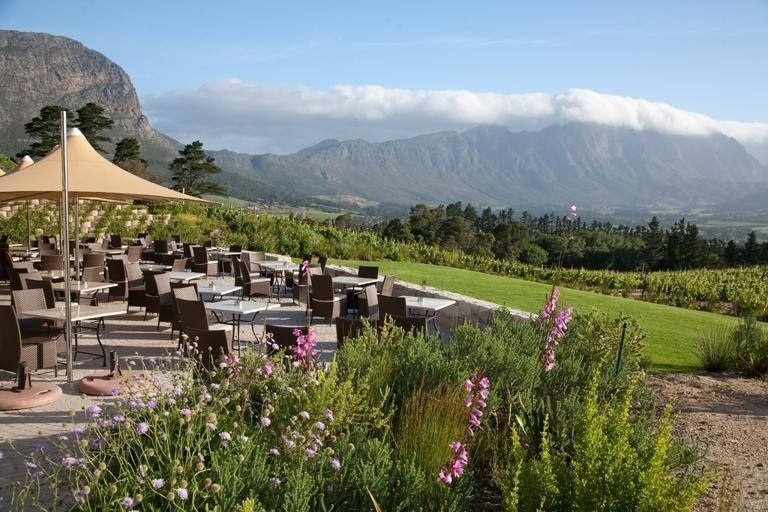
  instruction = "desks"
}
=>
[397,295,456,339]
